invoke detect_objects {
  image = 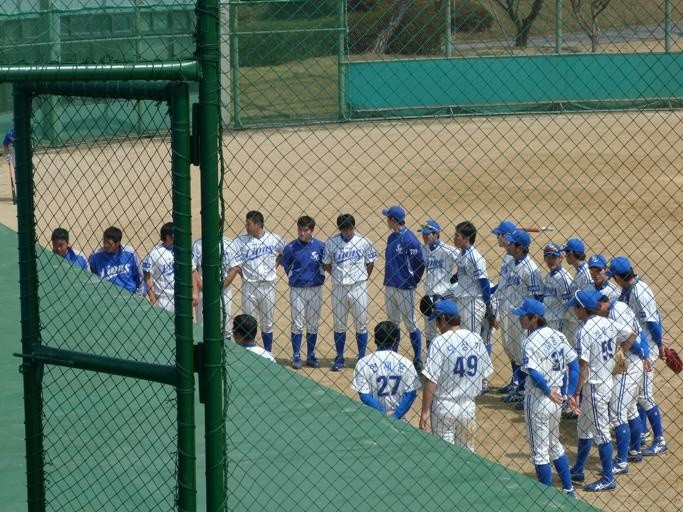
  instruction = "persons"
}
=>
[512,298,576,500]
[2,114,15,161]
[141,221,199,316]
[380,205,544,410]
[419,300,494,452]
[193,210,378,370]
[350,322,423,421]
[86,226,147,295]
[50,227,90,271]
[542,238,668,491]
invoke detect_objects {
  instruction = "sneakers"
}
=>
[569,430,667,492]
[307,356,319,367]
[413,358,424,375]
[483,381,489,392]
[564,410,578,419]
[497,382,524,409]
[331,357,344,370]
[291,354,301,368]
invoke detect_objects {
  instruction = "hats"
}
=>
[382,206,406,222]
[492,221,531,249]
[543,239,632,309]
[512,299,547,317]
[426,300,459,321]
[417,220,441,234]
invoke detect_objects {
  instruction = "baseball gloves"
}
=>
[611,345,628,376]
[665,348,683,374]
